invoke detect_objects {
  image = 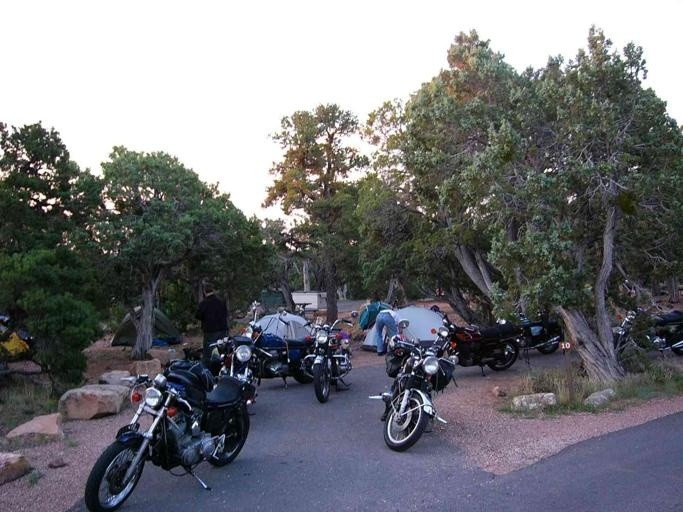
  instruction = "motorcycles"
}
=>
[430,304,524,377]
[368,319,457,452]
[83,358,257,512]
[499,303,563,354]
[612,279,683,360]
[206,300,353,403]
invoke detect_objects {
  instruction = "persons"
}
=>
[429,304,440,312]
[194,283,228,369]
[375,309,399,356]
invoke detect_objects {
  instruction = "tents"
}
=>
[0,315,35,362]
[110,306,181,347]
[359,306,445,350]
